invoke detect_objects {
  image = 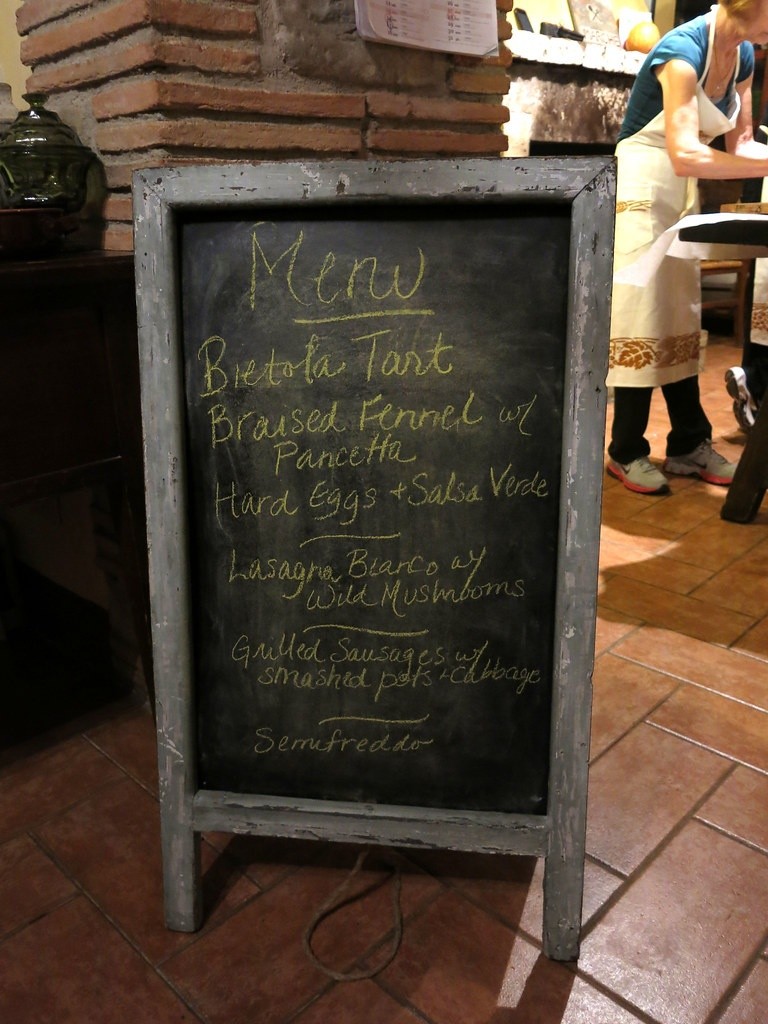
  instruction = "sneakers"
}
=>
[607,445,669,493]
[665,436,736,483]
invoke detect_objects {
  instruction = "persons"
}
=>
[605,0,768,493]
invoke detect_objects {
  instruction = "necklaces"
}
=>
[715,41,734,89]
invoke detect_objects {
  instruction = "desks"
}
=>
[677,213,767,525]
[0,249,156,729]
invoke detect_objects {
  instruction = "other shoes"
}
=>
[725,366,758,427]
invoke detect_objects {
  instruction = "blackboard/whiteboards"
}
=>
[135,155,619,854]
